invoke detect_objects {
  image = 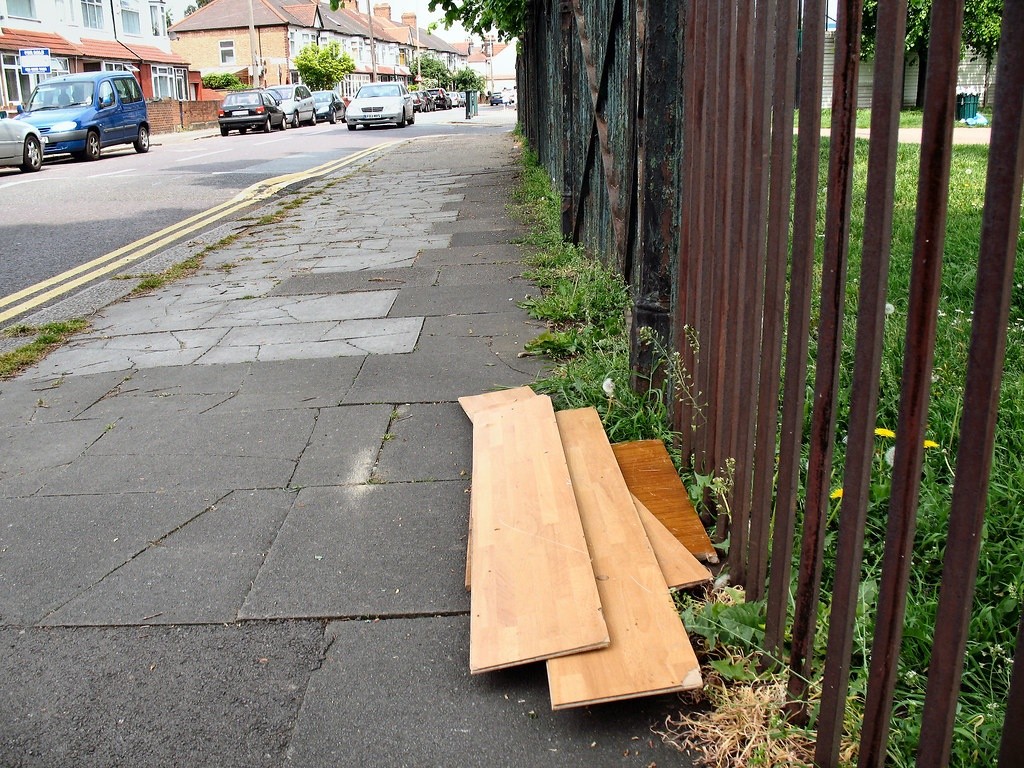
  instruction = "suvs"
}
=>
[427,87,452,110]
[264,84,318,129]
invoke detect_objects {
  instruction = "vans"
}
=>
[11,71,151,162]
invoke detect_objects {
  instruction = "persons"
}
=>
[500,87,512,110]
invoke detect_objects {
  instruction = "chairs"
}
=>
[58,94,70,104]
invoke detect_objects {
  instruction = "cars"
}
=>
[0,110,45,173]
[217,89,287,137]
[490,91,514,106]
[408,89,437,113]
[346,82,414,130]
[445,92,466,108]
[310,90,347,124]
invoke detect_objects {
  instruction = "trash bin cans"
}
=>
[955,92,981,121]
[480,94,486,104]
